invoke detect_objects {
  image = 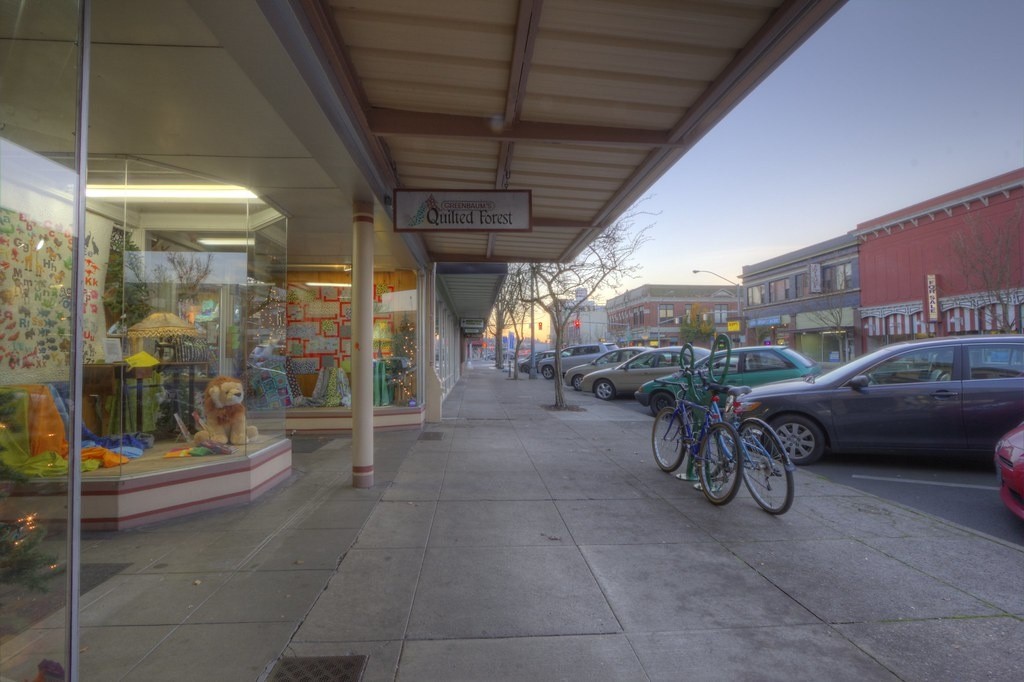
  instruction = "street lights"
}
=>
[657,290,675,348]
[692,269,742,348]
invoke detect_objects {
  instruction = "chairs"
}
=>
[927,351,953,381]
[746,354,762,369]
[658,354,679,367]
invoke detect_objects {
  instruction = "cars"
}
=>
[580,345,754,402]
[519,350,573,374]
[724,333,1024,468]
[489,352,515,360]
[565,347,683,392]
[995,421,1024,519]
[518,352,549,369]
[634,345,825,419]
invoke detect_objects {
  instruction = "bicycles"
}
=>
[679,362,797,516]
[651,379,743,506]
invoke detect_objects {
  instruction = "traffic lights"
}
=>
[539,322,542,329]
[576,320,580,329]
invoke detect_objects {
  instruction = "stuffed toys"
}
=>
[194,375,259,445]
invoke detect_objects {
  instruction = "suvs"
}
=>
[538,342,619,380]
